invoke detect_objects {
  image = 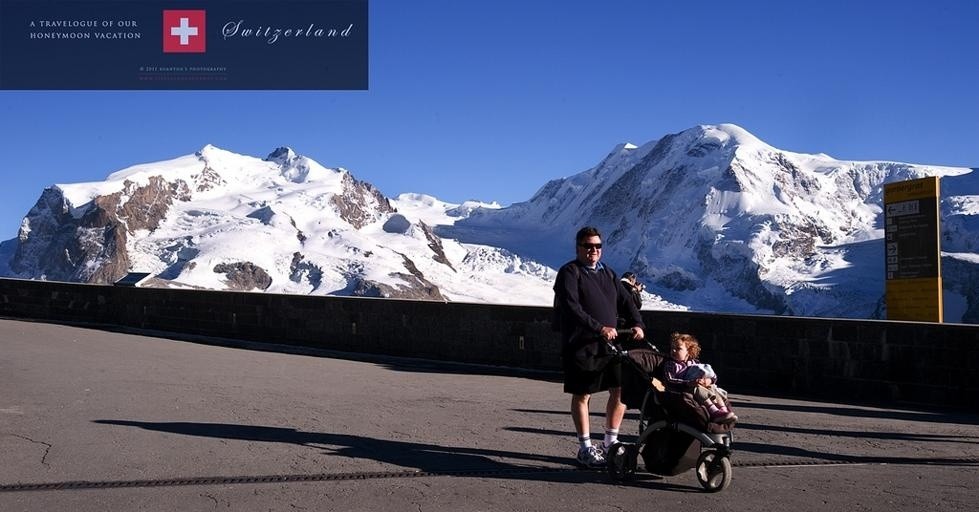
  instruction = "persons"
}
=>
[615,271,647,345]
[661,329,739,424]
[551,226,649,473]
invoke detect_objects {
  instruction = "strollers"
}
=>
[603,328,737,493]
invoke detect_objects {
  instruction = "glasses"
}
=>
[578,243,602,248]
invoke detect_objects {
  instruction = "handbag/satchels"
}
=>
[685,364,714,380]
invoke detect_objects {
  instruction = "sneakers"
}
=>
[598,440,625,457]
[577,444,608,468]
[710,410,737,424]
